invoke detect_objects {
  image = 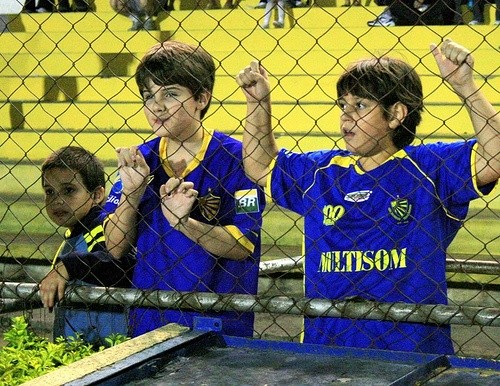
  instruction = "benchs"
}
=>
[0,0,500,285]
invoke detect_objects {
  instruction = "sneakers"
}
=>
[367,12,395,26]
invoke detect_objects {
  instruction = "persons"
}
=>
[100,42,266,338]
[37,147,136,349]
[21,0,499,30]
[237,38,500,356]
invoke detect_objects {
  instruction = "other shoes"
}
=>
[262,24,269,28]
[131,21,144,31]
[144,20,156,30]
[493,20,500,24]
[468,20,483,24]
[294,0,303,7]
[273,21,284,28]
[254,2,267,8]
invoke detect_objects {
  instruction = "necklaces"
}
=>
[170,167,186,173]
